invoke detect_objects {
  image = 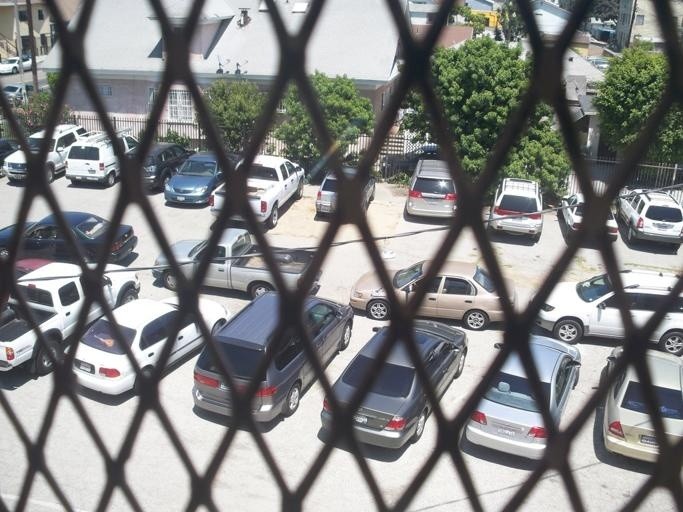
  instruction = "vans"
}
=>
[186,287,355,429]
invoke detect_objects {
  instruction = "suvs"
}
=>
[63,126,141,188]
[0,123,89,186]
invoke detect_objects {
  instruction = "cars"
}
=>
[0,82,32,105]
[346,254,520,333]
[0,52,37,75]
[313,312,469,453]
[0,137,19,174]
[164,150,239,207]
[310,169,376,218]
[142,141,197,194]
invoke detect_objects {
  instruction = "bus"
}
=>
[377,143,439,180]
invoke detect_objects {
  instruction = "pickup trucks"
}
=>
[148,226,325,297]
[205,152,307,230]
